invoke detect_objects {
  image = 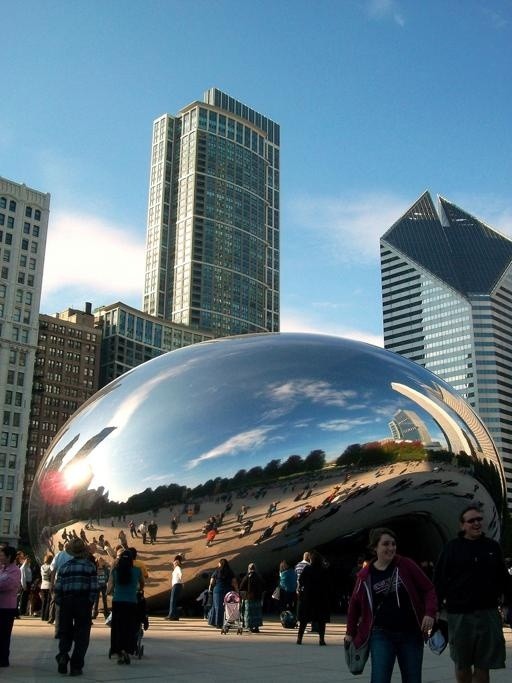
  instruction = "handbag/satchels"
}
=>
[429,599,449,656]
[240,591,253,599]
[272,588,282,600]
[280,611,298,629]
[343,637,370,674]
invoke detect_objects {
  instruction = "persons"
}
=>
[48,537,148,677]
[2,545,21,666]
[46,508,158,578]
[168,551,337,645]
[167,461,498,546]
[16,550,59,624]
[344,507,512,683]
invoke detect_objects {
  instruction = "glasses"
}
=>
[465,516,483,524]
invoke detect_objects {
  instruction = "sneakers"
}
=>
[58,658,82,677]
[248,628,259,633]
[297,639,326,645]
[118,649,129,664]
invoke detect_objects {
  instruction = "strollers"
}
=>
[221,590,244,634]
